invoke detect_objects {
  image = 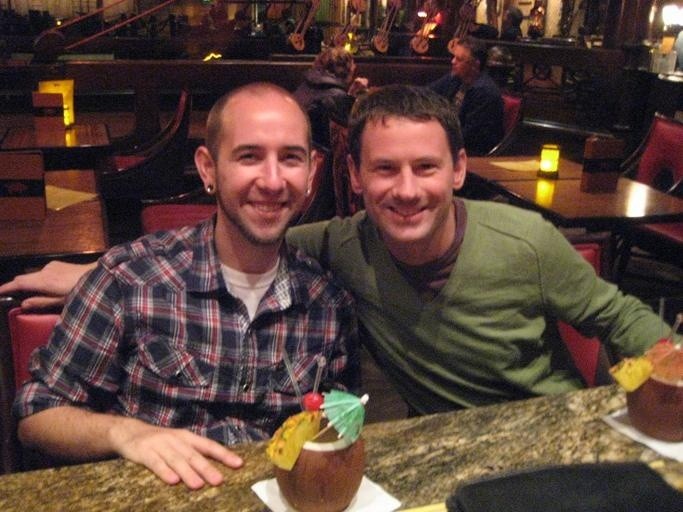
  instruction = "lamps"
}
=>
[660,5,683,59]
[418,3,433,20]
[537,144,562,179]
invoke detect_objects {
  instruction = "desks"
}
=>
[1,390,683,511]
[467,156,683,231]
[1,168,111,263]
[1,119,109,151]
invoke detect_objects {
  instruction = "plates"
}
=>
[251,477,400,511]
[604,408,683,464]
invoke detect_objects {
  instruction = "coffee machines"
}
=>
[648,30,677,75]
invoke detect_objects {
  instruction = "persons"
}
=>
[0,83,683,419]
[424,36,508,200]
[292,48,369,220]
[496,6,524,40]
[9,80,361,490]
[115,7,325,60]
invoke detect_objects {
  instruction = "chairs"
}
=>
[485,90,528,153]
[616,221,683,290]
[617,114,682,192]
[554,243,612,385]
[100,86,191,218]
[6,305,61,410]
[134,142,326,237]
[326,113,359,217]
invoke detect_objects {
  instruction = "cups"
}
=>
[33,93,64,130]
[580,137,622,193]
[607,314,683,441]
[266,357,369,511]
